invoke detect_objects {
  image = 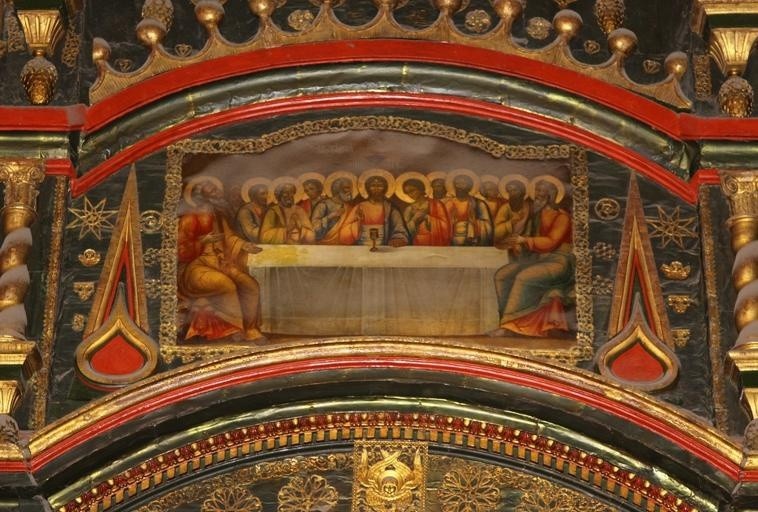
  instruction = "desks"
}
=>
[243,239,510,337]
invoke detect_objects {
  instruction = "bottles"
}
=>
[716,69,755,118]
[19,51,58,104]
